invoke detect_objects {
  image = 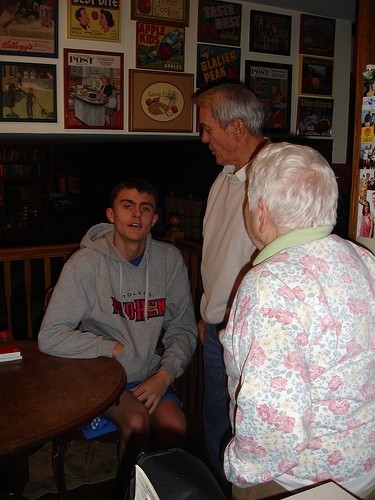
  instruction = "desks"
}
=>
[0,340,129,461]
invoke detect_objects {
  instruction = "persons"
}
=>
[364,145,375,165]
[359,192,375,238]
[194,83,272,478]
[365,111,375,127]
[223,142,375,500]
[365,173,370,180]
[37,178,198,466]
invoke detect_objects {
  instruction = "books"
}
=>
[0,330,22,362]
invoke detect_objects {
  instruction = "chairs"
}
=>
[0,244,123,500]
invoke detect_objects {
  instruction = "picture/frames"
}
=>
[0,0,337,138]
